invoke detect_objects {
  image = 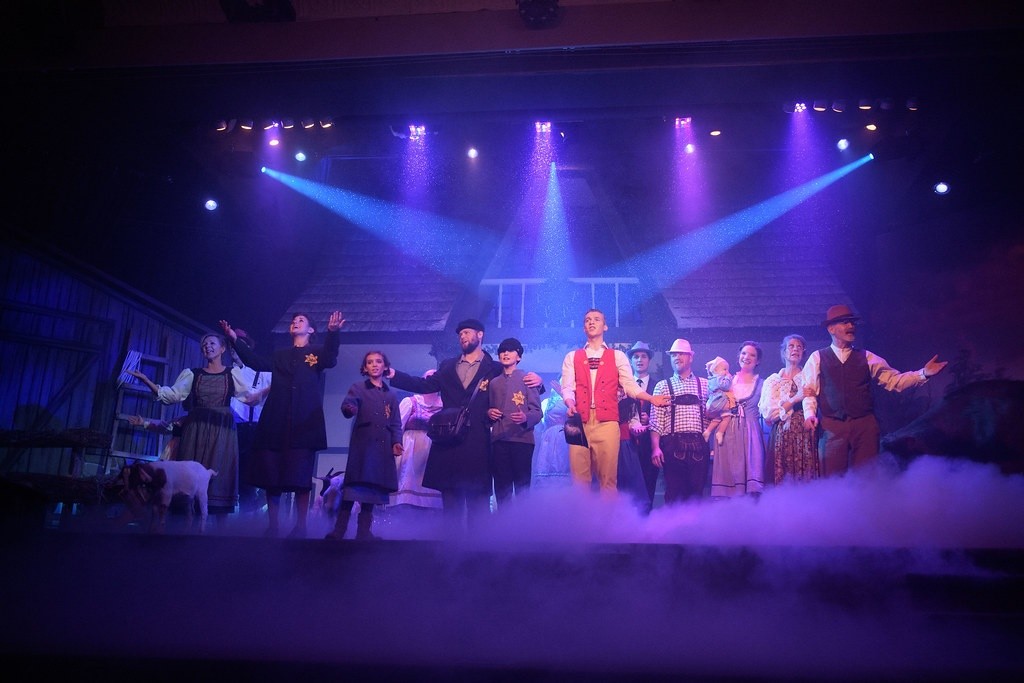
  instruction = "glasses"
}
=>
[508,348,520,355]
[841,319,856,324]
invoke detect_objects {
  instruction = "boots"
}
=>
[355,514,381,540]
[326,511,350,540]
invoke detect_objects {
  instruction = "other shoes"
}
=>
[287,525,308,539]
[263,528,279,537]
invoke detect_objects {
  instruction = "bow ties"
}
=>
[679,375,693,381]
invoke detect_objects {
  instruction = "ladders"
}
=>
[105,323,172,477]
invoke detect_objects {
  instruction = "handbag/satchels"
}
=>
[426,408,471,448]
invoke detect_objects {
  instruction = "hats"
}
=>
[497,339,524,356]
[455,319,485,334]
[666,339,695,356]
[226,328,254,348]
[627,340,655,358]
[564,412,589,448]
[822,305,854,326]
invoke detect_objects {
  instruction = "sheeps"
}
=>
[114,456,218,534]
[319,468,346,495]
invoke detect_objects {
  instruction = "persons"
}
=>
[128,415,187,461]
[627,341,660,500]
[218,311,345,538]
[702,356,734,446]
[758,334,818,486]
[801,305,949,480]
[225,328,272,528]
[384,369,443,509]
[549,380,651,514]
[649,339,710,506]
[324,351,404,538]
[487,338,543,512]
[561,309,672,499]
[711,341,765,496]
[384,319,546,535]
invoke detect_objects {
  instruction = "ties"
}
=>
[637,379,643,386]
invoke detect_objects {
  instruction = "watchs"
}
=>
[126,333,271,534]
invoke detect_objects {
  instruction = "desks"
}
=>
[0,437,110,482]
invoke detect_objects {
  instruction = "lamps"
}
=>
[213,116,334,131]
[813,95,920,113]
